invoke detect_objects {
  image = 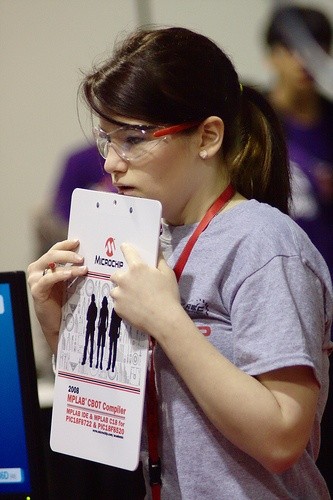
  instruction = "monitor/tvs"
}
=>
[0,270,49,500]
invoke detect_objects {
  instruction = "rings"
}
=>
[42,267,48,276]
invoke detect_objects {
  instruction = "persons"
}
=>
[24,27,331,500]
[34,3,333,278]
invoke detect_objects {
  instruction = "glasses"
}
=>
[93,121,195,160]
